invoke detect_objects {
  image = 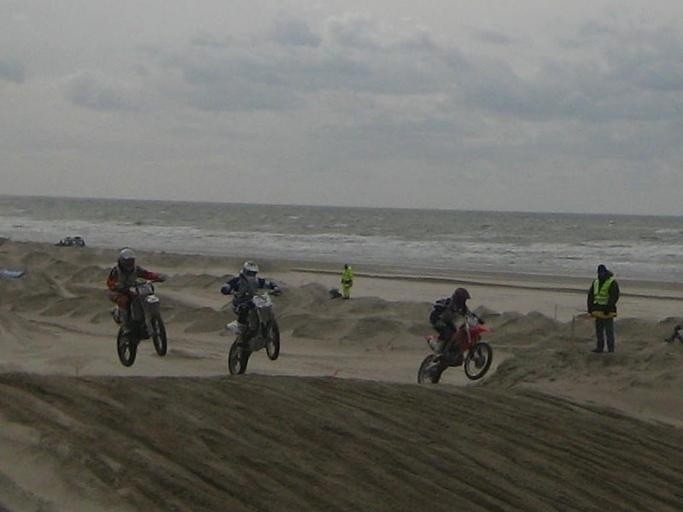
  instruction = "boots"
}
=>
[119,309,132,334]
[591,341,615,352]
[432,340,445,358]
[236,322,246,345]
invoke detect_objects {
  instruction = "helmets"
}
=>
[119,249,136,273]
[452,288,470,306]
[242,261,259,276]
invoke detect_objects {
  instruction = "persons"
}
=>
[429,286,485,359]
[220,260,282,334]
[587,264,620,354]
[339,263,353,299]
[104,248,168,325]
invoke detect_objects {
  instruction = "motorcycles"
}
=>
[110,279,167,367]
[419,311,492,386]
[225,290,281,375]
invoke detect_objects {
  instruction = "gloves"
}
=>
[221,284,232,295]
[273,287,282,296]
[157,275,166,282]
[478,319,484,324]
[449,305,457,312]
[117,283,127,292]
